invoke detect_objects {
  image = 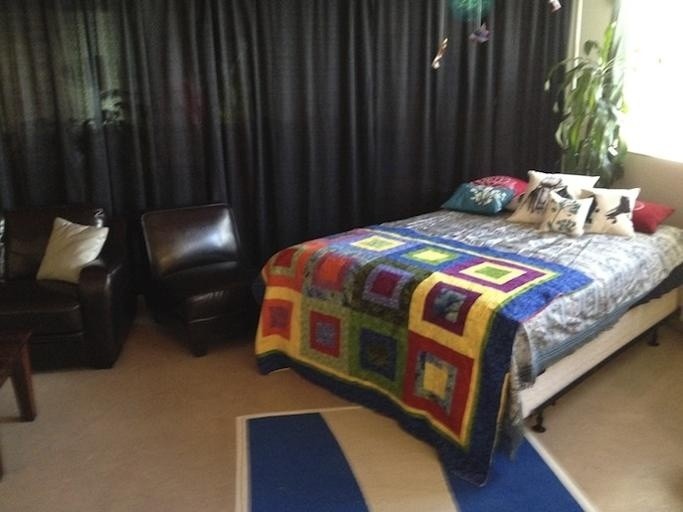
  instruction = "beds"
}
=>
[251,149,682,486]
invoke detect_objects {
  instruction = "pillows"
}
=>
[580,187,641,237]
[537,191,594,237]
[506,166,597,224]
[630,199,678,234]
[36,218,109,285]
[439,173,526,215]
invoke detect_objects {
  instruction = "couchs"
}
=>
[135,200,258,359]
[0,207,130,369]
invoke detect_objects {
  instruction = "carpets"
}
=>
[234,403,598,512]
